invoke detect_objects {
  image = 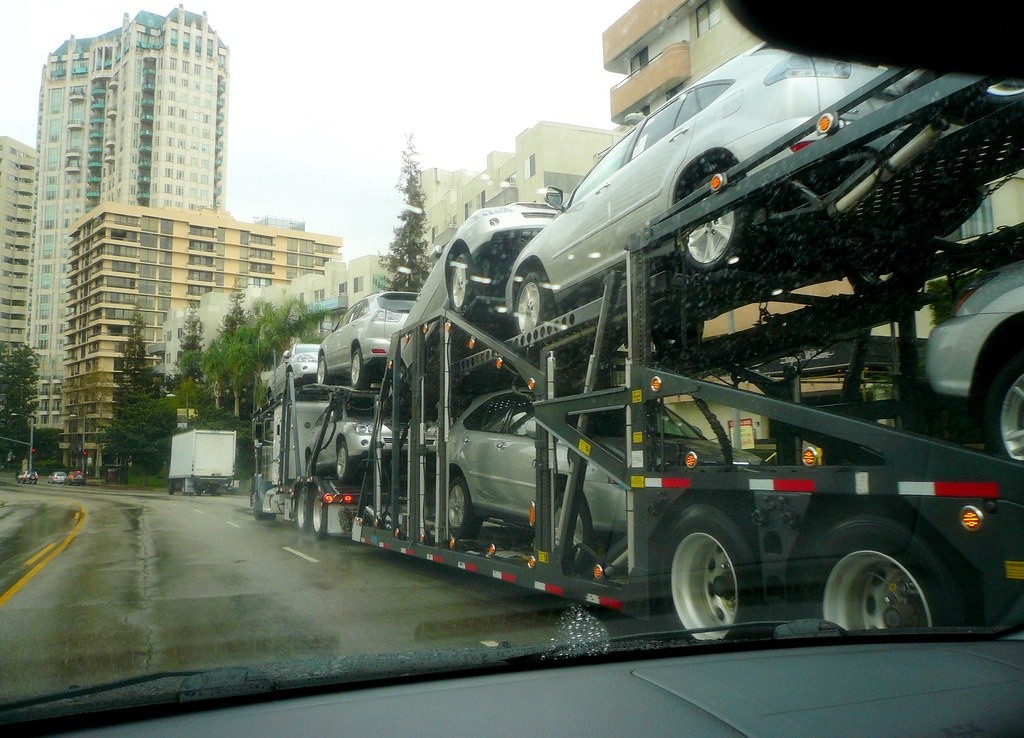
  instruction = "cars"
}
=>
[386,201,559,388]
[317,292,418,392]
[48,471,67,484]
[503,41,941,334]
[304,414,436,484]
[441,386,767,565]
[64,471,86,487]
[925,260,1024,466]
[265,343,321,404]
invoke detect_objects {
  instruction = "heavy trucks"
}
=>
[249,63,1024,643]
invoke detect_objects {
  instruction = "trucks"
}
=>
[167,428,236,496]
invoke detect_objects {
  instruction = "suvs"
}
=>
[18,470,38,485]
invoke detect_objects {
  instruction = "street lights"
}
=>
[70,414,85,472]
[11,412,34,472]
